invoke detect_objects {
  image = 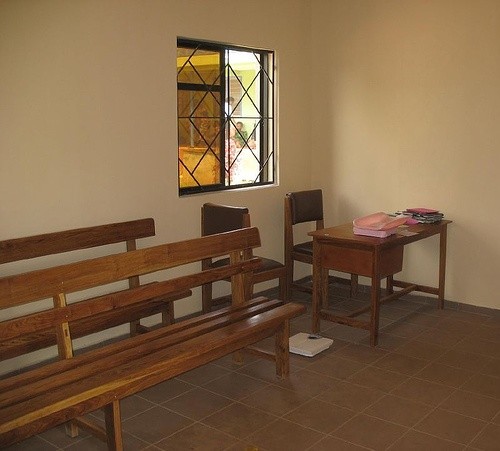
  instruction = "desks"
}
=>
[307,220,453,347]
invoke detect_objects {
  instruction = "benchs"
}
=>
[0,218,193,361]
[0,226,306,451]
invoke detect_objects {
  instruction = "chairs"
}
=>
[201,202,287,314]
[284,190,358,310]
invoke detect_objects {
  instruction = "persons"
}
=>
[233,122,248,149]
[225,96,236,140]
[185,109,220,148]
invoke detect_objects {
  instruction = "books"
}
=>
[395,208,445,223]
